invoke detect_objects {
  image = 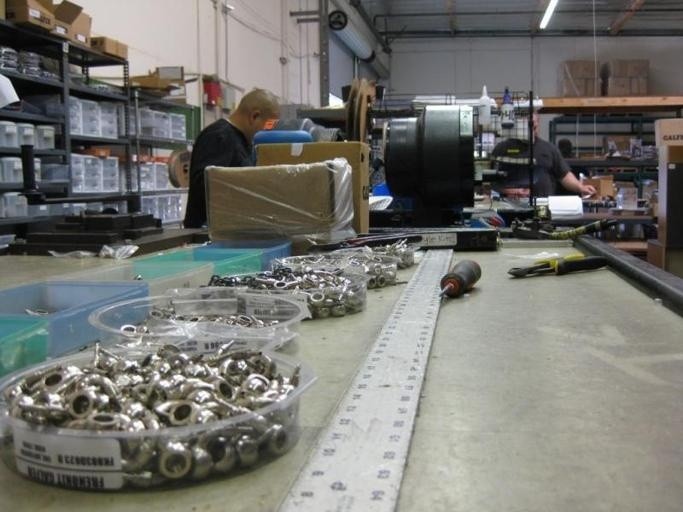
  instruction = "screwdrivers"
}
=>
[438,260,482,298]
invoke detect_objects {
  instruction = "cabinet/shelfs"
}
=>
[1,19,194,229]
[548,117,659,212]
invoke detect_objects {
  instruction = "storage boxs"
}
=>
[560,58,651,99]
[0,0,94,47]
[203,141,371,256]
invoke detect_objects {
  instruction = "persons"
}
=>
[488,115,597,201]
[553,139,592,199]
[179,88,281,229]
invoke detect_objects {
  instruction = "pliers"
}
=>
[508,254,609,277]
[308,234,423,251]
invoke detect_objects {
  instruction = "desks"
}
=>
[1,238,683,512]
